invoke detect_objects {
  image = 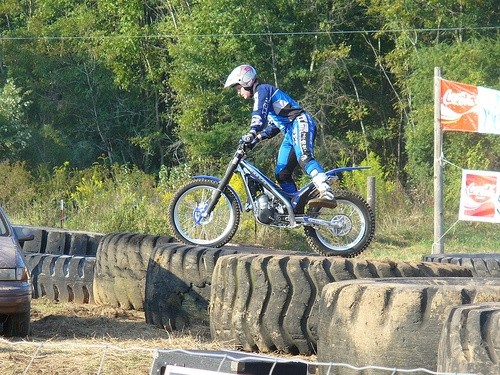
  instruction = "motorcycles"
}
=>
[168,136,375,259]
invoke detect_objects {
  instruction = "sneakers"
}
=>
[308,188,337,209]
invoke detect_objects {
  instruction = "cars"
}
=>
[0,206,36,340]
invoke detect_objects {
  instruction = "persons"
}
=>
[223,65,338,210]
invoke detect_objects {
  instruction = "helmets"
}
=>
[223,65,257,87]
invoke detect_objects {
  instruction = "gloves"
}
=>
[242,130,257,143]
[251,137,259,145]
